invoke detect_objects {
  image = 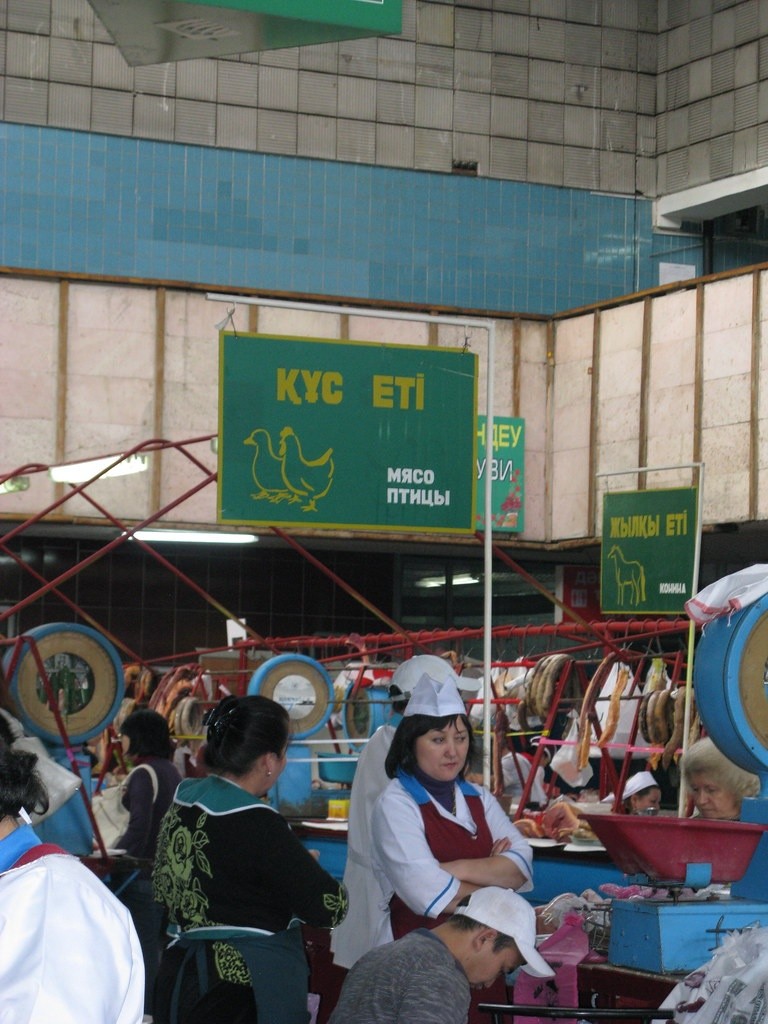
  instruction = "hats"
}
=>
[621,771,659,800]
[454,886,556,978]
[389,655,481,703]
[402,674,468,717]
[529,736,556,762]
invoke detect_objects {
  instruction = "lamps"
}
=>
[48,454,148,484]
[0,477,29,494]
[122,526,256,545]
[415,572,481,588]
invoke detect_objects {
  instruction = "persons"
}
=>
[107,655,759,1024]
[0,718,148,1023]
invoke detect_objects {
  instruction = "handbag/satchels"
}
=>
[0,708,82,824]
[88,763,158,849]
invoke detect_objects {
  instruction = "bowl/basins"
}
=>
[577,814,768,883]
[315,751,359,783]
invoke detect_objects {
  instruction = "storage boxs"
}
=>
[317,753,359,783]
[578,814,767,882]
